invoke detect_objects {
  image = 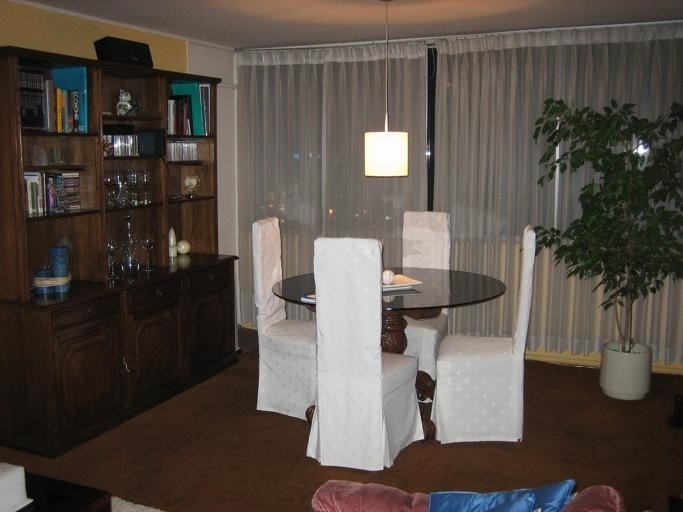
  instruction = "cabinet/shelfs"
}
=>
[0,46,223,303]
[0,254,240,458]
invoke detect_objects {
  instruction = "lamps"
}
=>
[365,0,409,177]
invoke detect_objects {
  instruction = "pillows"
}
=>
[428,479,576,512]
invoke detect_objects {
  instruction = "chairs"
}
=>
[250,216,317,423]
[402,210,450,382]
[306,236,426,472]
[430,225,538,444]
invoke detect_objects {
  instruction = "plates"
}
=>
[382,274,424,292]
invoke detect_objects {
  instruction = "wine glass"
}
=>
[104,239,119,281]
[105,168,155,209]
[141,231,157,273]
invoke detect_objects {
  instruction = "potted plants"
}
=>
[532,95,683,401]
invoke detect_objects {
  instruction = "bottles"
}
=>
[121,215,140,276]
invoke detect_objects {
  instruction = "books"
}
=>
[13,66,211,218]
[300,291,316,302]
[381,273,423,289]
[381,286,413,293]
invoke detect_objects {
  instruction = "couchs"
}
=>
[310,479,623,512]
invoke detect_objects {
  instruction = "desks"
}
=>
[17,471,112,512]
